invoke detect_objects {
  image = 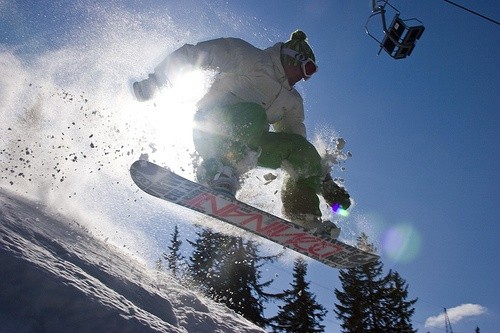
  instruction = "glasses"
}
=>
[283,47,317,80]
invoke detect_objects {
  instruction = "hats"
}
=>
[281,28,315,65]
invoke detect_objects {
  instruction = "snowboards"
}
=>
[130,160,380,270]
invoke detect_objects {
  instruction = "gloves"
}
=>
[321,175,351,211]
[132,76,158,101]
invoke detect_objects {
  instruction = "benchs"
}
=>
[380,14,424,59]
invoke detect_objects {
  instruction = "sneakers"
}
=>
[292,213,340,239]
[196,164,243,197]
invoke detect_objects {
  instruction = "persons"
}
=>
[133,29,350,238]
